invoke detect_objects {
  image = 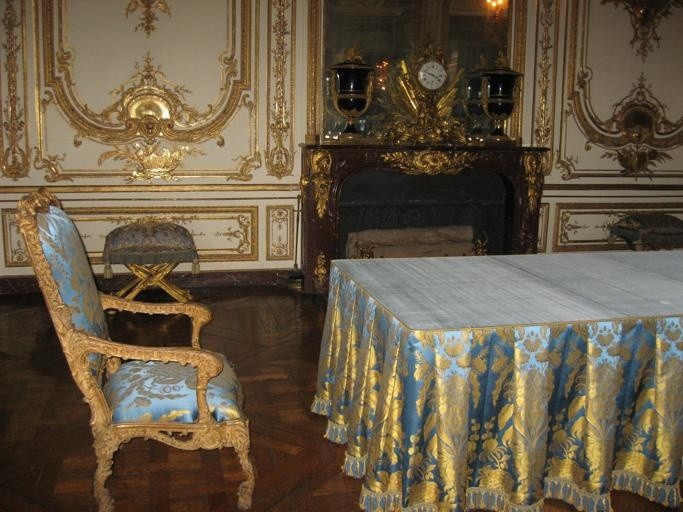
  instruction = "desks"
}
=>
[293,142,551,298]
[299,243,682,511]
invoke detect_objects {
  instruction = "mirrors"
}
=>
[305,1,527,136]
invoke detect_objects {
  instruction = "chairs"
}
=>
[12,181,263,512]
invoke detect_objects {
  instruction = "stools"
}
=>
[606,208,682,249]
[96,220,202,308]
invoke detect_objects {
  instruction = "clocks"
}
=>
[414,57,451,93]
